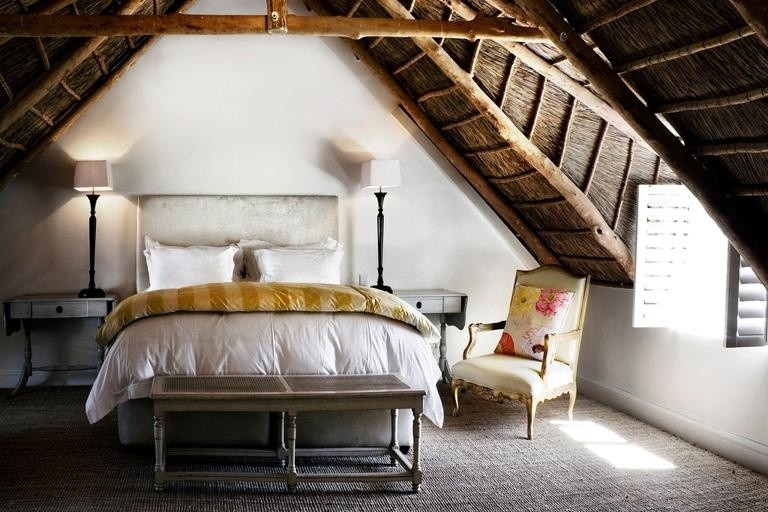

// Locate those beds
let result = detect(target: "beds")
[118,194,417,456]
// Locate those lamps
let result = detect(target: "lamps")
[71,161,115,298]
[360,159,403,295]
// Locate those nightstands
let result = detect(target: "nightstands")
[390,288,468,393]
[6,293,116,396]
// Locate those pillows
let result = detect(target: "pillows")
[142,234,344,291]
[493,280,577,366]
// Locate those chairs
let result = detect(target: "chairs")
[449,262,592,441]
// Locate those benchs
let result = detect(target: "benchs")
[149,374,427,494]
